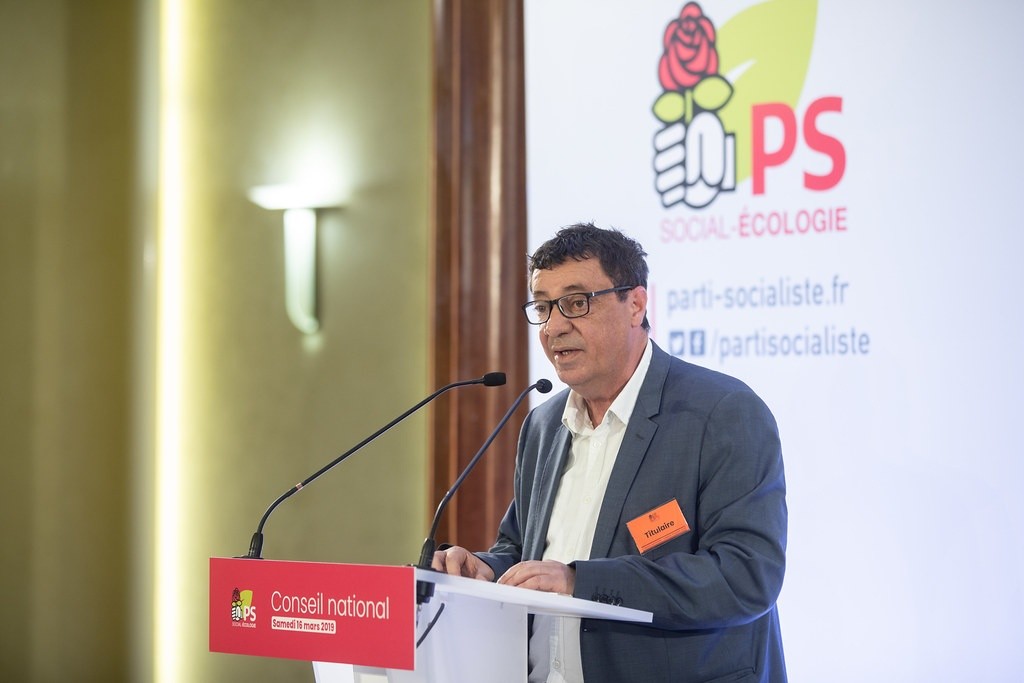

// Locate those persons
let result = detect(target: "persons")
[429,222,790,683]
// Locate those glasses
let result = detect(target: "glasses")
[522,286,632,323]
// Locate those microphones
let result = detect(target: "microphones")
[407,378,552,574]
[247,371,506,553]
